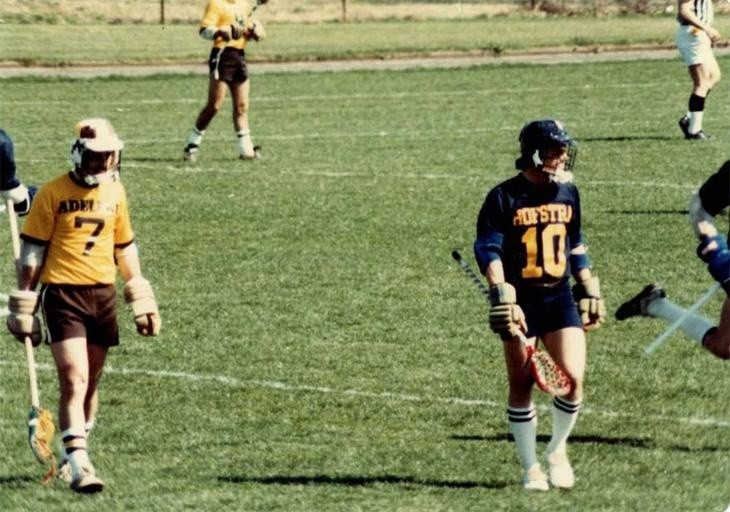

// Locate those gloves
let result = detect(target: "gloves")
[124,277,161,336]
[572,277,606,332]
[218,23,242,40]
[696,236,730,299]
[489,283,528,342]
[7,291,42,347]
[245,20,265,41]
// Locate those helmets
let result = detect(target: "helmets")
[515,119,578,183]
[70,118,123,184]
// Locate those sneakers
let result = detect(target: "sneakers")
[679,115,711,140]
[56,461,74,483]
[524,462,549,492]
[544,447,574,489]
[240,146,265,160]
[616,284,665,320]
[72,462,104,493]
[184,144,198,162]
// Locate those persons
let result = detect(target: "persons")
[615,160,730,360]
[473,118,607,493]
[675,0,723,142]
[0,128,38,218]
[183,0,266,162]
[6,117,162,494]
